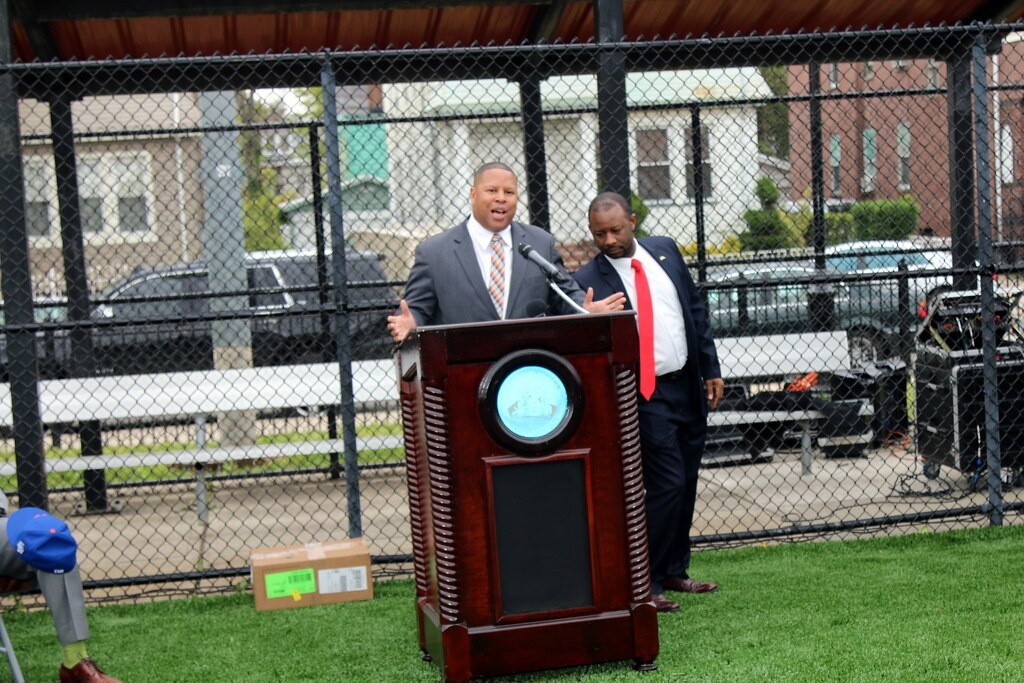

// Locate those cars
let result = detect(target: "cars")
[706,241,1001,371]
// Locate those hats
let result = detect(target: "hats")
[6,506,78,575]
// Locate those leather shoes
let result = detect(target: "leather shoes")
[59,656,122,683]
[669,570,718,593]
[652,595,681,613]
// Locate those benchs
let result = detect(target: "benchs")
[0,329,874,525]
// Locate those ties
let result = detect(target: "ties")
[629,259,657,401]
[487,233,508,321]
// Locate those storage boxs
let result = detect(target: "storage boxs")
[249,537,373,612]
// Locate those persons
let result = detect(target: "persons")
[0,489,122,683]
[570,193,723,612]
[387,163,626,342]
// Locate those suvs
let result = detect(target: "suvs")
[1,247,403,377]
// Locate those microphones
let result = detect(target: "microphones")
[517,242,564,280]
